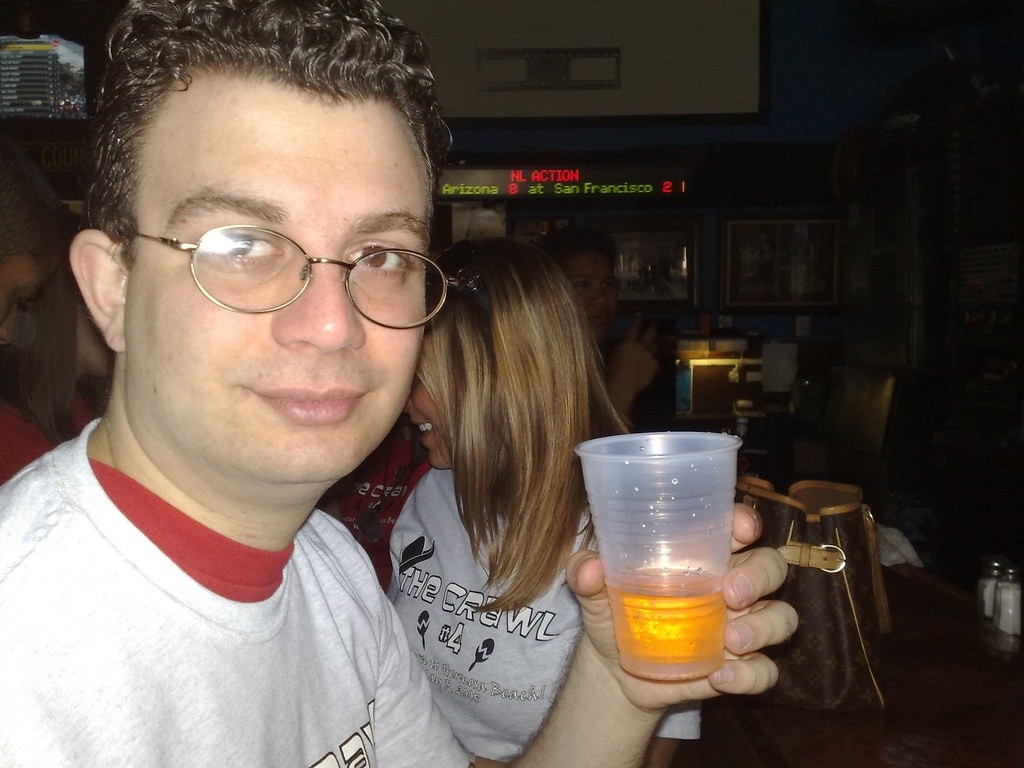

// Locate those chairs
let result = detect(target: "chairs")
[815,365,903,488]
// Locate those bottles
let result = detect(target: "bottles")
[978,562,1001,619]
[994,569,1024,640]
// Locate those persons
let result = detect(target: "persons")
[0,1,801,768]
[1,135,702,768]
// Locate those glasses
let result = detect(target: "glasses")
[112,223,477,330]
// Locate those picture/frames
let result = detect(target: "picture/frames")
[588,214,703,316]
[717,210,847,315]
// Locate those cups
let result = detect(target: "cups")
[574,432,743,679]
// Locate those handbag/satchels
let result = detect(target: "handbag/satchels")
[734,474,895,723]
